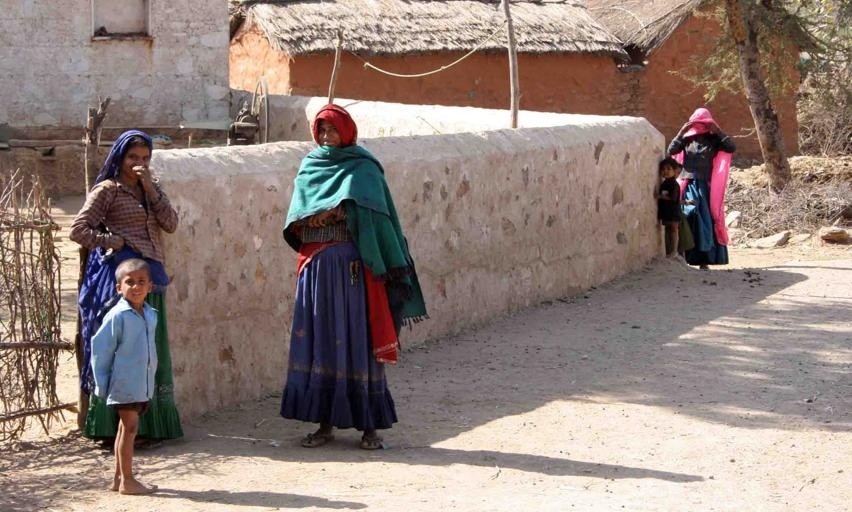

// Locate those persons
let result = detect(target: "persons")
[89,257,161,496]
[280,103,429,451]
[653,157,681,259]
[666,107,736,271]
[68,128,185,446]
[667,161,694,254]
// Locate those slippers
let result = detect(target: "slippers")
[360,435,382,449]
[302,429,334,447]
